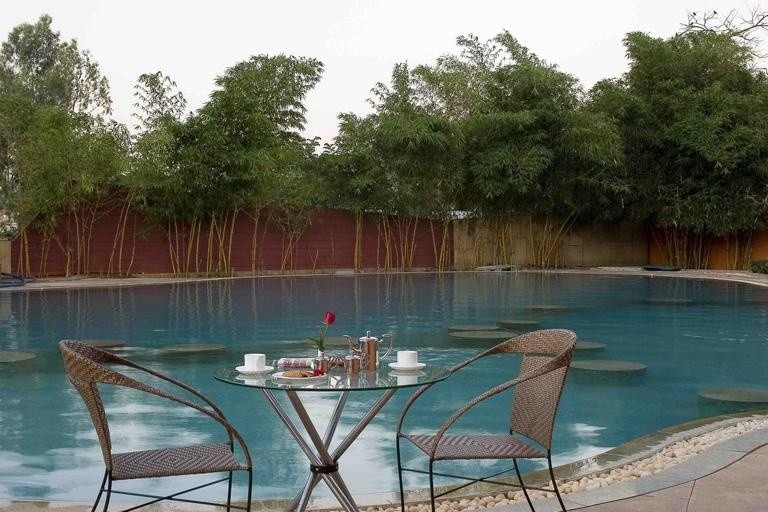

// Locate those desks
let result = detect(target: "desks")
[214,356,452,512]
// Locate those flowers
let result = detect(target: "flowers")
[309,309,337,349]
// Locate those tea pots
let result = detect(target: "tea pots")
[311,353,338,375]
[364,368,381,388]
[341,331,393,371]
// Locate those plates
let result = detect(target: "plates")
[387,372,427,378]
[271,378,328,386]
[233,364,275,378]
[269,370,329,380]
[235,373,275,382]
[388,362,426,372]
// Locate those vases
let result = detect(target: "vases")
[317,350,324,362]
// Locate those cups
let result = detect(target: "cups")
[243,378,269,389]
[345,370,361,388]
[397,350,418,366]
[397,376,419,386]
[242,353,267,370]
[343,354,361,376]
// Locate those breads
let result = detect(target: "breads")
[280,371,312,377]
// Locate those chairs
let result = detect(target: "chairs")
[397,327,579,512]
[58,338,255,512]
